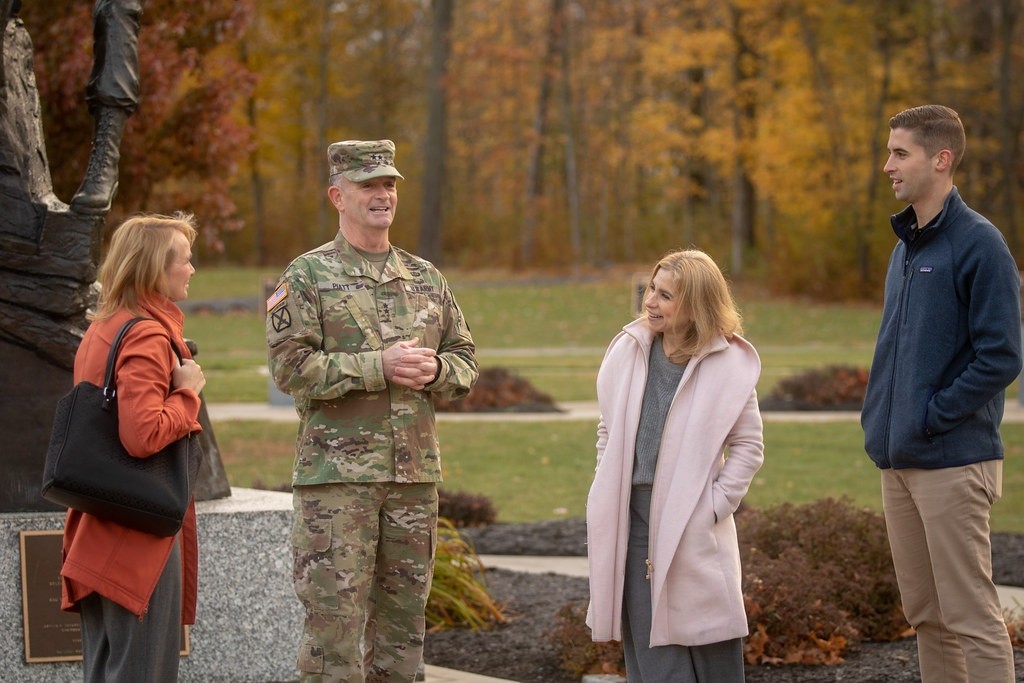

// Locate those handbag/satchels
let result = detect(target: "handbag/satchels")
[40,318,203,537]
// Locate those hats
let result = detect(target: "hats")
[327,140,404,182]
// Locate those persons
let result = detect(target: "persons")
[585,243,767,683]
[60,210,208,683]
[859,103,1023,683]
[264,138,480,683]
